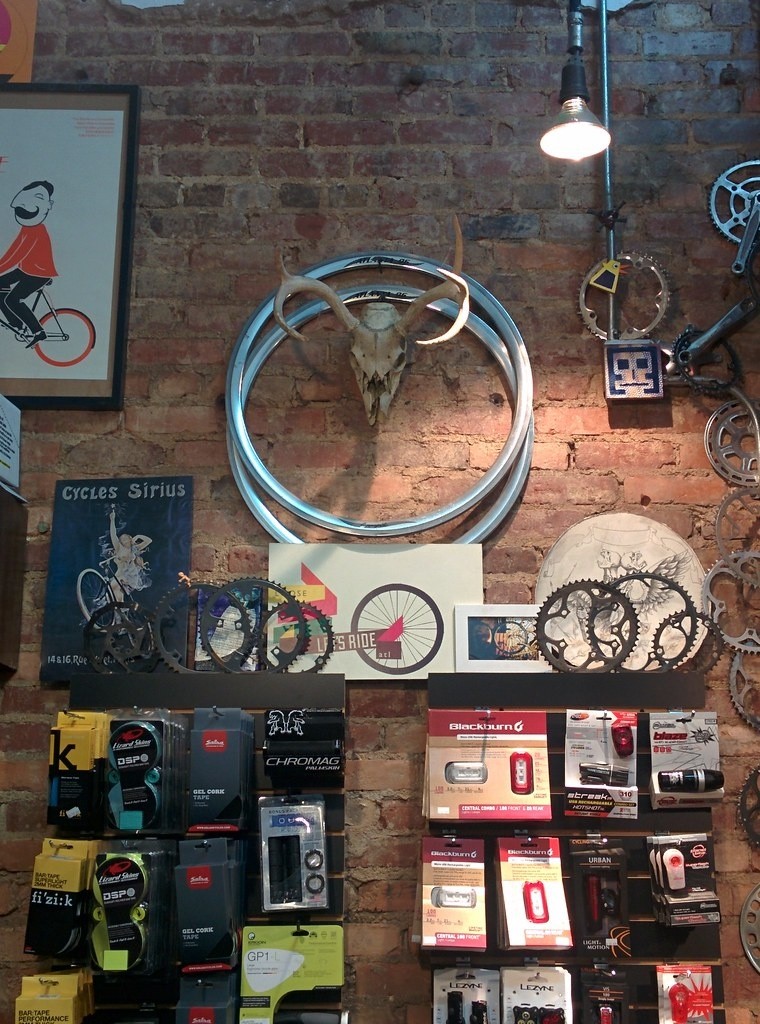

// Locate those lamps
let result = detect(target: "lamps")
[540,0,610,159]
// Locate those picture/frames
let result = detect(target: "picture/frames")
[454,604,552,673]
[0,80,143,411]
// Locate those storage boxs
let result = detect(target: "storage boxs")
[14,708,344,1024]
